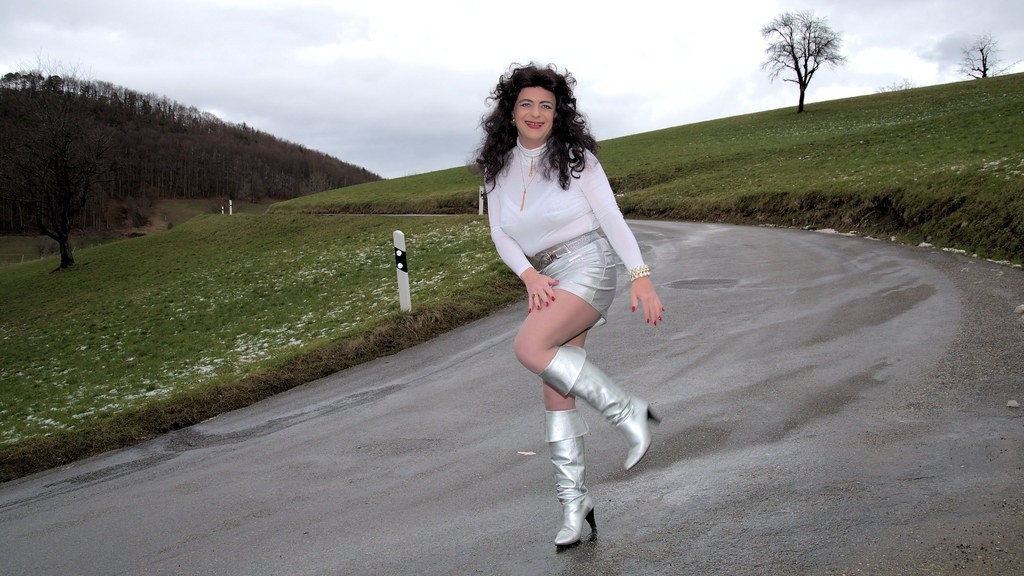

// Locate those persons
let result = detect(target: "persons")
[468,63,666,545]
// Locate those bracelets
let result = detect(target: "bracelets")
[628,265,650,282]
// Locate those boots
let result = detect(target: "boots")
[537,344,662,471]
[543,408,596,546]
